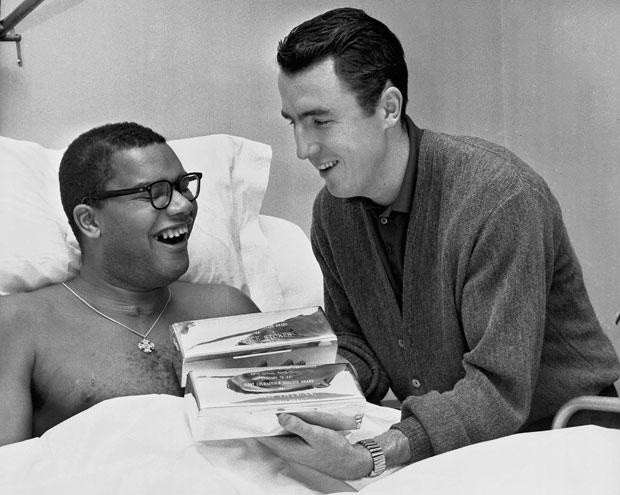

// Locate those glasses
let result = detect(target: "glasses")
[91,170,202,210]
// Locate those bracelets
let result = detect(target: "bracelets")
[358,438,387,479]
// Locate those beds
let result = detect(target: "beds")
[4,210,620,494]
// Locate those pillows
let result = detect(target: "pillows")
[2,129,281,317]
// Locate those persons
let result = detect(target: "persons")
[0,118,261,450]
[253,5,619,482]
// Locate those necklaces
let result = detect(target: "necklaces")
[61,280,174,355]
